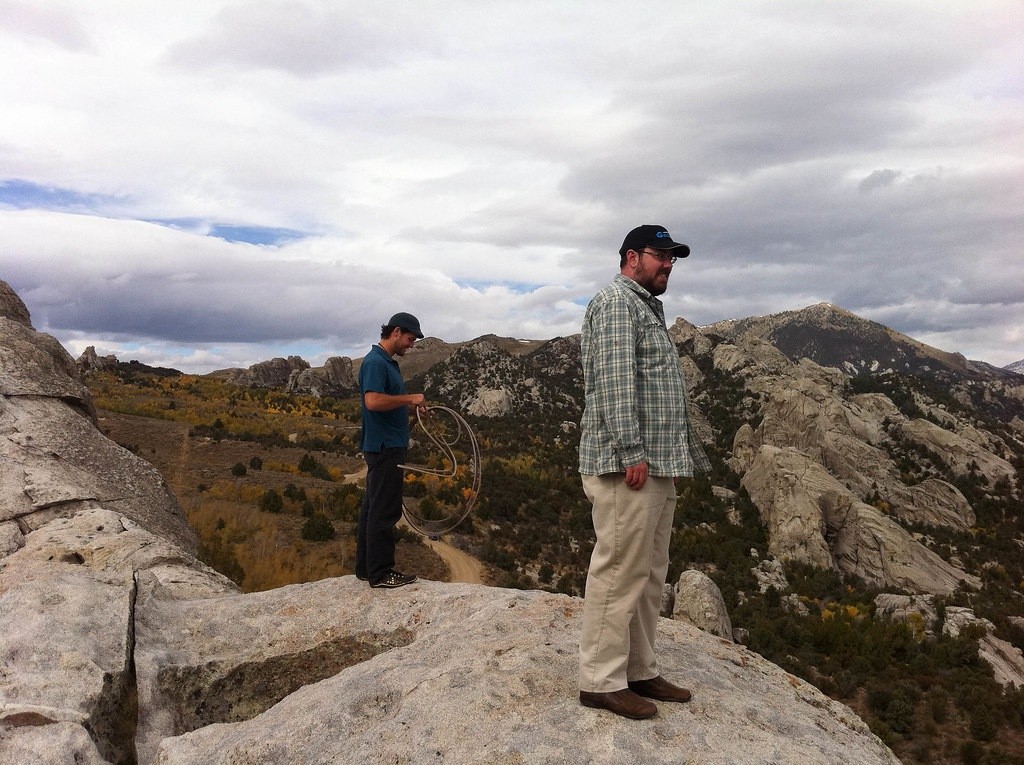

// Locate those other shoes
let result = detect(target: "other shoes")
[579,687,658,720]
[628,675,691,703]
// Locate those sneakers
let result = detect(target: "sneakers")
[355,571,370,579]
[370,569,418,588]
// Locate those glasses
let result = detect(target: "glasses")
[636,250,677,265]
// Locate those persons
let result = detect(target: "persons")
[578,224,712,720]
[355,312,426,588]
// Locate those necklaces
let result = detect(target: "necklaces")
[378,342,387,353]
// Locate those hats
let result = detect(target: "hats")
[619,224,690,258]
[388,312,424,338]
